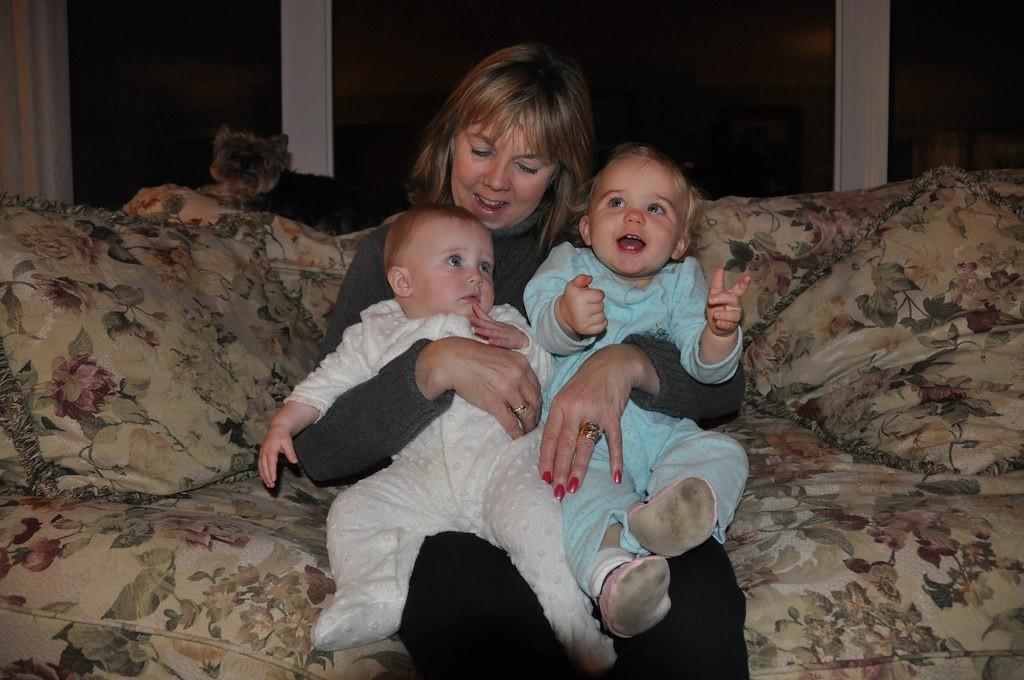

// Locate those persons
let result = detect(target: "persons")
[256,43,753,680]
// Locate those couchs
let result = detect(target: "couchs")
[0,184,1024,680]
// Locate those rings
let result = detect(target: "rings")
[578,420,605,444]
[512,399,530,416]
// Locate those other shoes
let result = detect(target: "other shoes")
[627,475,717,558]
[598,555,672,637]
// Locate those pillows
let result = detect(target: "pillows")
[738,166,1024,477]
[124,187,378,332]
[0,192,327,505]
